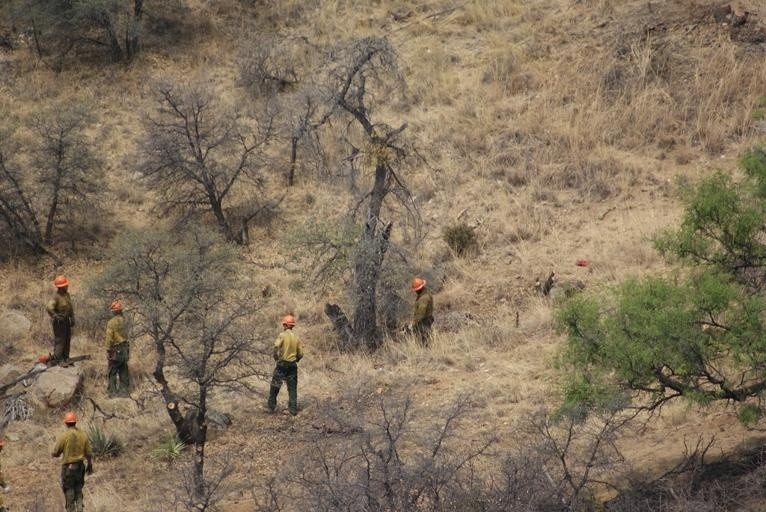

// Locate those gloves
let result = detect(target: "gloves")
[54,313,64,320]
[86,463,92,473]
[69,318,75,327]
[2,486,10,493]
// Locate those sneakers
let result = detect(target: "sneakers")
[58,358,73,368]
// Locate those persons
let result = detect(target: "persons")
[51,412,93,512]
[409,278,434,348]
[105,301,130,399]
[263,315,304,416]
[46,275,75,367]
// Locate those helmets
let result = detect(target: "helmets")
[52,276,70,288]
[282,315,296,325]
[110,300,123,311]
[411,277,426,291]
[64,412,76,423]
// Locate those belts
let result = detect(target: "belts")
[106,351,112,360]
[281,360,296,363]
[64,460,83,465]
